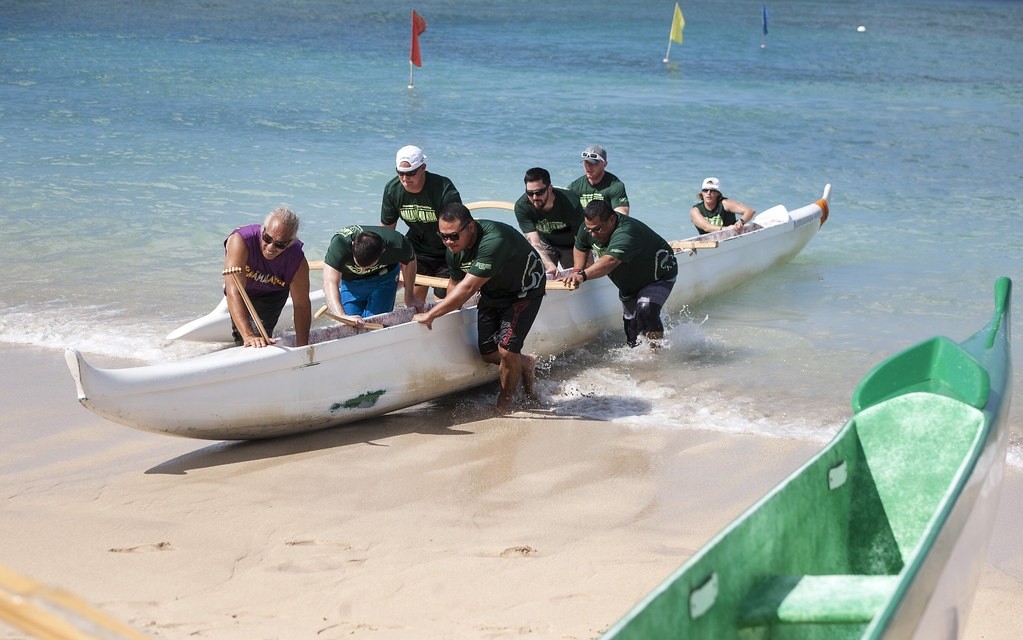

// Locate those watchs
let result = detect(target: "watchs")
[578,270,587,281]
[739,219,745,225]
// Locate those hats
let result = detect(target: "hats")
[702,177,725,198]
[580,145,607,163]
[395,145,428,172]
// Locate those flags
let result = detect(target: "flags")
[761,6,767,35]
[670,3,685,44]
[411,9,426,67]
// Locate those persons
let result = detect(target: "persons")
[558,200,678,344]
[223,208,311,347]
[412,203,545,404]
[567,145,630,257]
[380,146,462,305]
[324,225,423,331]
[514,167,587,280]
[691,176,756,235]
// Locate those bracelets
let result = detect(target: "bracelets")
[573,269,580,272]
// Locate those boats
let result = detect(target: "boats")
[165,201,515,348]
[61,181,837,445]
[592,271,1017,640]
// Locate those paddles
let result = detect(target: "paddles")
[314,303,387,331]
[221,266,273,346]
[753,204,790,226]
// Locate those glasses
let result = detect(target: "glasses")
[526,184,550,197]
[262,226,292,249]
[436,220,472,241]
[581,152,605,163]
[396,164,423,176]
[586,220,609,232]
[701,188,719,193]
